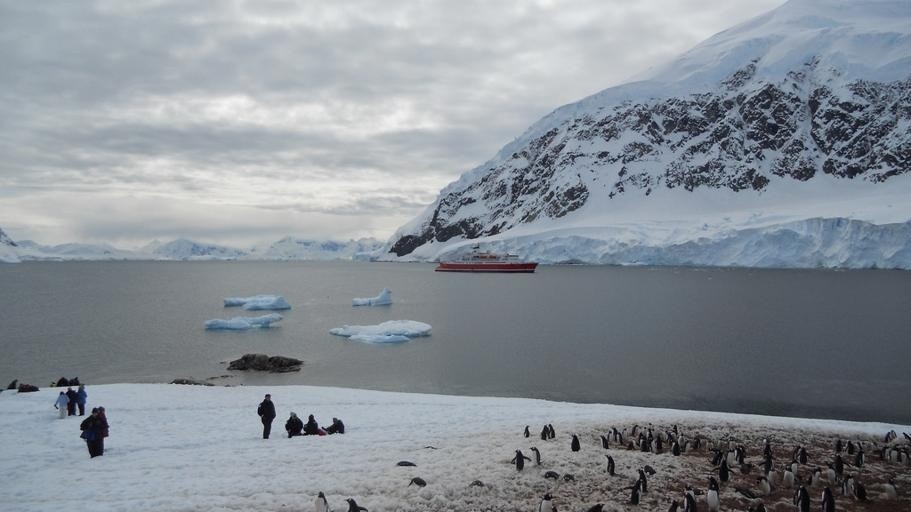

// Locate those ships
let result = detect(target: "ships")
[433,242,539,273]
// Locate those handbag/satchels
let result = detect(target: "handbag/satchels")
[80,431,95,440]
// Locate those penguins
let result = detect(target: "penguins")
[344,497,369,512]
[314,491,331,512]
[408,477,426,486]
[507,424,911,512]
[397,461,418,466]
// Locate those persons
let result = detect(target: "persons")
[55,386,109,458]
[258,394,276,439]
[285,412,344,438]
